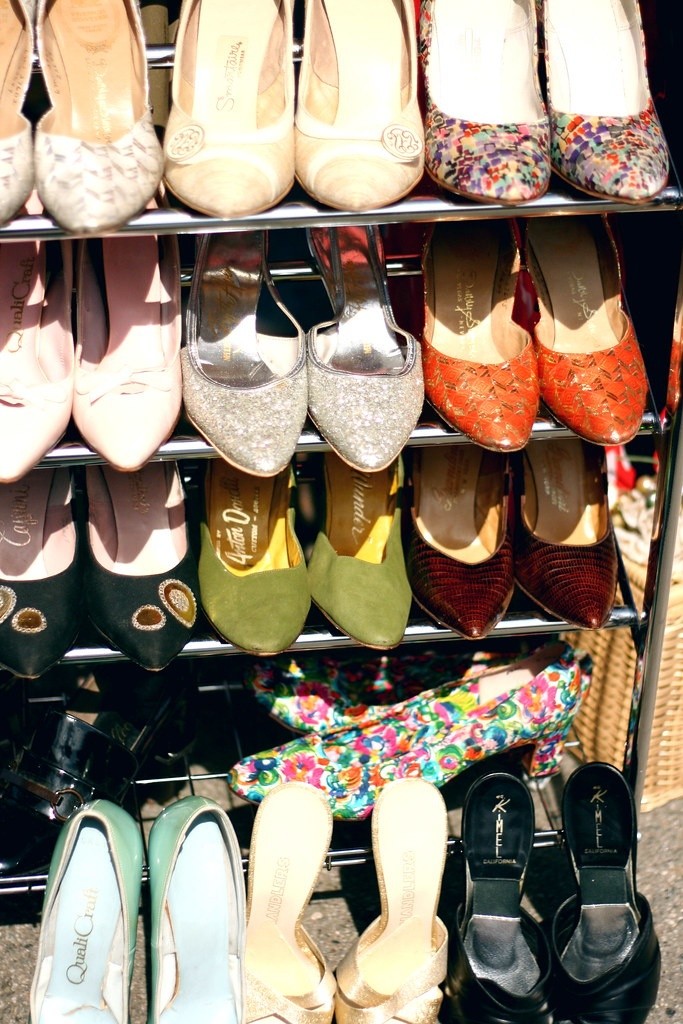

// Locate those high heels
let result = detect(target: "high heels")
[424,0,667,203]
[0,645,660,1023]
[0,235,183,482]
[182,226,424,478]
[424,213,648,452]
[1,0,163,233]
[162,0,423,216]
[0,436,618,678]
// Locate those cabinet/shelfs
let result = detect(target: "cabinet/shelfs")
[0,45,683,895]
